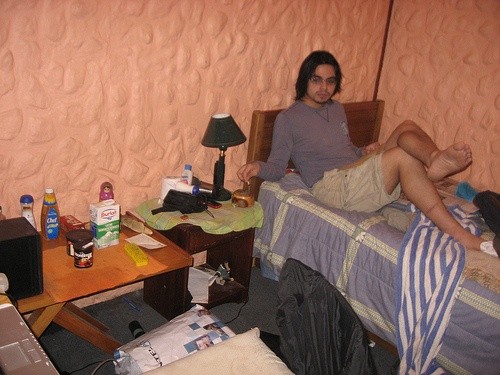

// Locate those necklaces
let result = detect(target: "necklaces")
[309,102,330,124]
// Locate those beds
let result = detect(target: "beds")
[243,100,500,375]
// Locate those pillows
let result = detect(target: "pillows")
[140,327,296,375]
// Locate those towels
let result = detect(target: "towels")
[393,203,485,375]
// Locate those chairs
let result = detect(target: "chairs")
[273,258,401,375]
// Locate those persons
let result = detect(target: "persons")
[196,306,228,352]
[237,50,488,251]
[99,182,114,203]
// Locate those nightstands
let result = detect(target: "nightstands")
[126,180,265,322]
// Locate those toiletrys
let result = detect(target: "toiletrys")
[183,163,195,188]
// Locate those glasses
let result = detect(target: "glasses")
[309,76,338,86]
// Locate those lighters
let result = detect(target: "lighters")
[207,200,222,208]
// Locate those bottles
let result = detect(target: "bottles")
[181,164,193,186]
[41,188,60,242]
[20,195,37,230]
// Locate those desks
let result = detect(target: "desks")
[15,211,194,352]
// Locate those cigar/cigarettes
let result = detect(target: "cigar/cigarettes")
[247,180,250,186]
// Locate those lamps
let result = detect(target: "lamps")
[201,113,247,201]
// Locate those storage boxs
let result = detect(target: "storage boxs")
[0,217,43,300]
